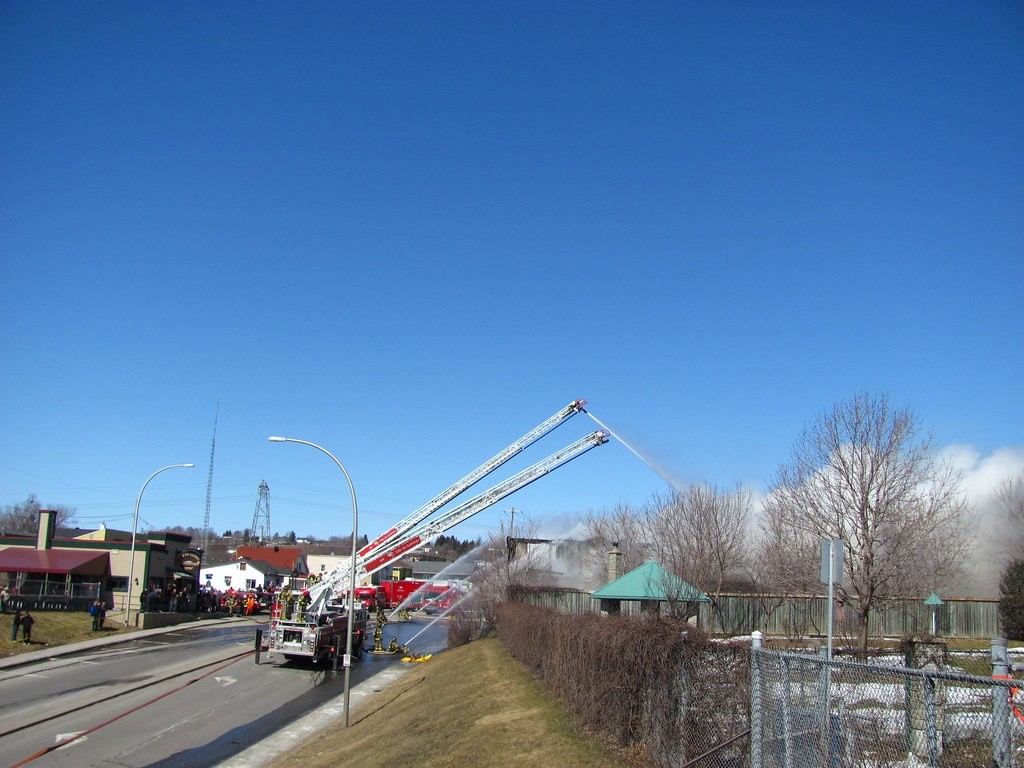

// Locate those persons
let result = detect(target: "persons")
[397,601,412,620]
[307,573,322,586]
[375,600,387,626]
[390,637,399,652]
[1,587,10,612]
[12,611,34,645]
[139,583,276,618]
[90,601,107,631]
[279,585,311,623]
[374,624,384,652]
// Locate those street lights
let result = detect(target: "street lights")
[125,464,195,626]
[268,436,358,728]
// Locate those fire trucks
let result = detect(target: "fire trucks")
[272,572,494,616]
[256,402,611,671]
